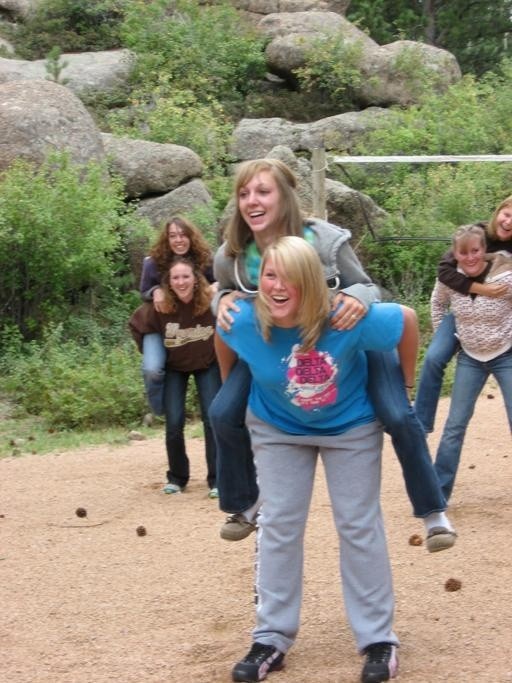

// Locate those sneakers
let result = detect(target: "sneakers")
[232,642,284,682]
[162,484,178,492]
[361,642,398,683]
[220,514,257,541]
[426,527,456,553]
[209,488,218,498]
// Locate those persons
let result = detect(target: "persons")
[425,225,510,516]
[412,195,512,434]
[137,214,236,415]
[214,233,418,683]
[215,154,457,553]
[128,257,228,499]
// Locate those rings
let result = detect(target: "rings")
[347,313,359,322]
